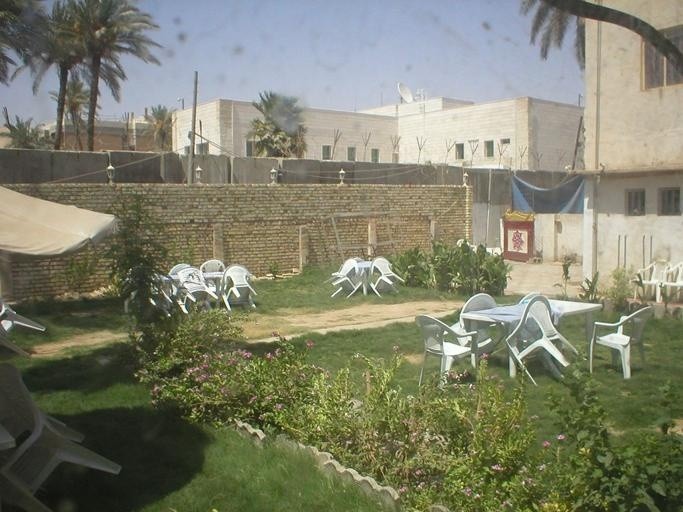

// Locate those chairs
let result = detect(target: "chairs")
[588,304,654,379]
[505,295,587,387]
[414,314,478,387]
[324,256,407,299]
[152,260,259,316]
[0,296,123,512]
[489,292,541,346]
[633,258,682,304]
[437,292,506,351]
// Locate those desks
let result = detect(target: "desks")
[461,299,603,378]
